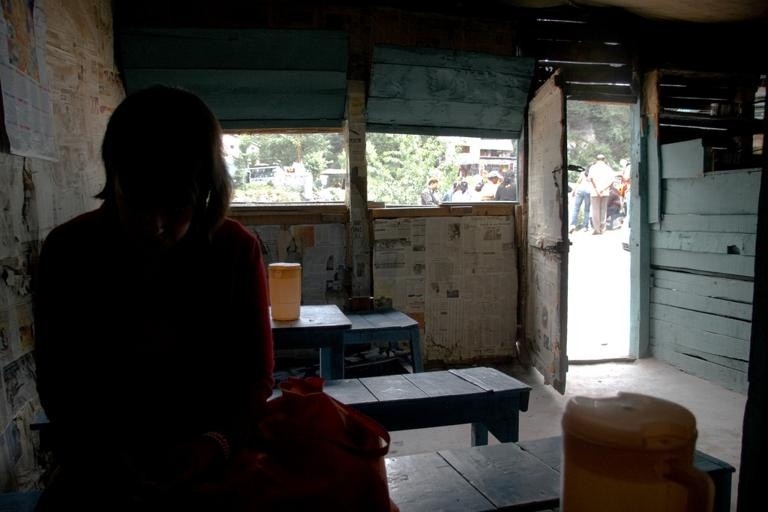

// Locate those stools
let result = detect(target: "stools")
[344,307,424,378]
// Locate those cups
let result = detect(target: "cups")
[560,389,716,512]
[268,262,302,321]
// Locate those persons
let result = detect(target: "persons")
[420,153,630,237]
[30,85,274,511]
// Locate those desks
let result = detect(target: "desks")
[268,303,352,380]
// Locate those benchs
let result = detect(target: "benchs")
[382,429,736,512]
[272,365,533,446]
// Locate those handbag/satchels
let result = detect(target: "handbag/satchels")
[225,378,398,512]
